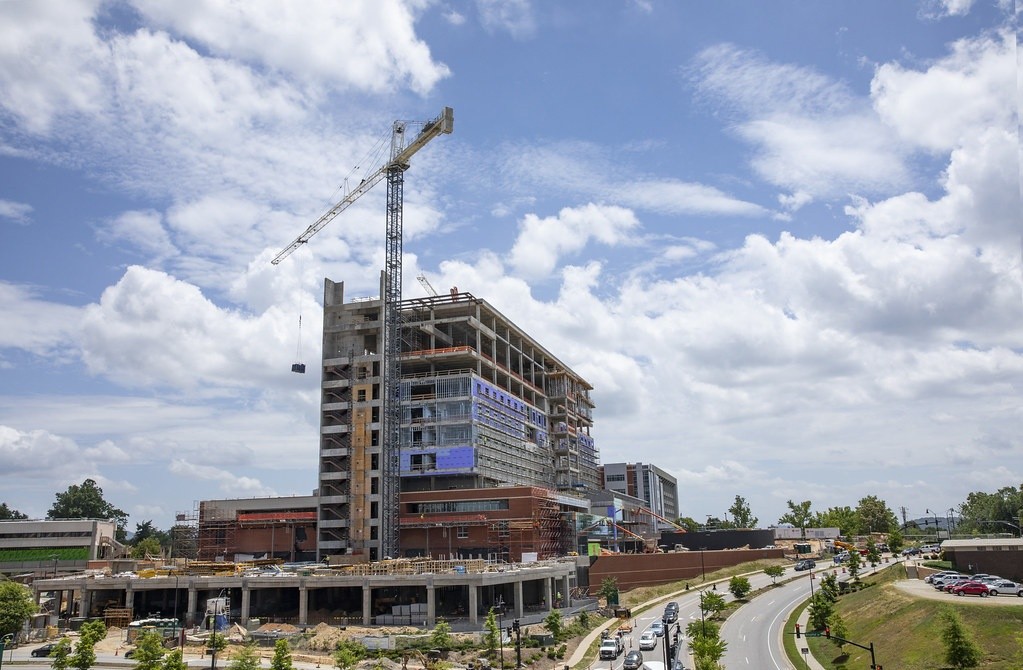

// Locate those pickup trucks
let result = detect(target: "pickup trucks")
[920,545,938,553]
[837,550,861,562]
[986,581,1023,597]
[858,549,869,556]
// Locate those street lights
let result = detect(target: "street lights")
[707,515,712,527]
[53,557,58,581]
[212,587,230,669]
[724,513,727,521]
[947,508,954,540]
[500,595,507,670]
[927,509,940,544]
[168,569,178,640]
[685,582,718,638]
[810,573,815,610]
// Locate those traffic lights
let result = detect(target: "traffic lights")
[507,628,513,638]
[878,665,882,670]
[513,622,517,631]
[825,627,831,639]
[795,624,800,639]
[516,620,519,630]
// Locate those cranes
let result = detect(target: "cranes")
[267,102,454,561]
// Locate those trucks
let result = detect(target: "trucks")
[598,636,626,659]
[659,543,690,552]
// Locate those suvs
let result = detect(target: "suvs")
[933,574,970,584]
[975,577,1001,583]
[954,582,989,597]
[970,574,989,579]
[624,650,643,669]
[925,571,959,582]
[638,630,657,650]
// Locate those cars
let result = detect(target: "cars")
[876,544,890,552]
[31,644,72,659]
[943,579,977,594]
[650,621,666,636]
[902,548,922,555]
[982,579,1010,584]
[935,581,954,591]
[662,611,677,623]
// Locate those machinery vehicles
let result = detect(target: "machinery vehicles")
[402,647,442,670]
[632,506,688,534]
[834,540,869,556]
[607,520,663,554]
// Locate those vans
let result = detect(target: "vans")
[795,559,815,571]
[664,602,680,613]
